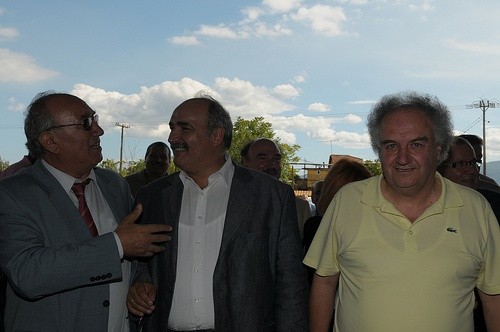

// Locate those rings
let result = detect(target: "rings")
[129,299,134,303]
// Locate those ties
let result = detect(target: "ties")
[72,178,99,238]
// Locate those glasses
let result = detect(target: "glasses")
[38,114,98,135]
[441,159,482,169]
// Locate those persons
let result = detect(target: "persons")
[0,89,173,332]
[240,138,311,240]
[437,135,500,226]
[0,151,37,179]
[301,90,500,332]
[301,158,372,270]
[124,141,171,199]
[125,90,311,332]
